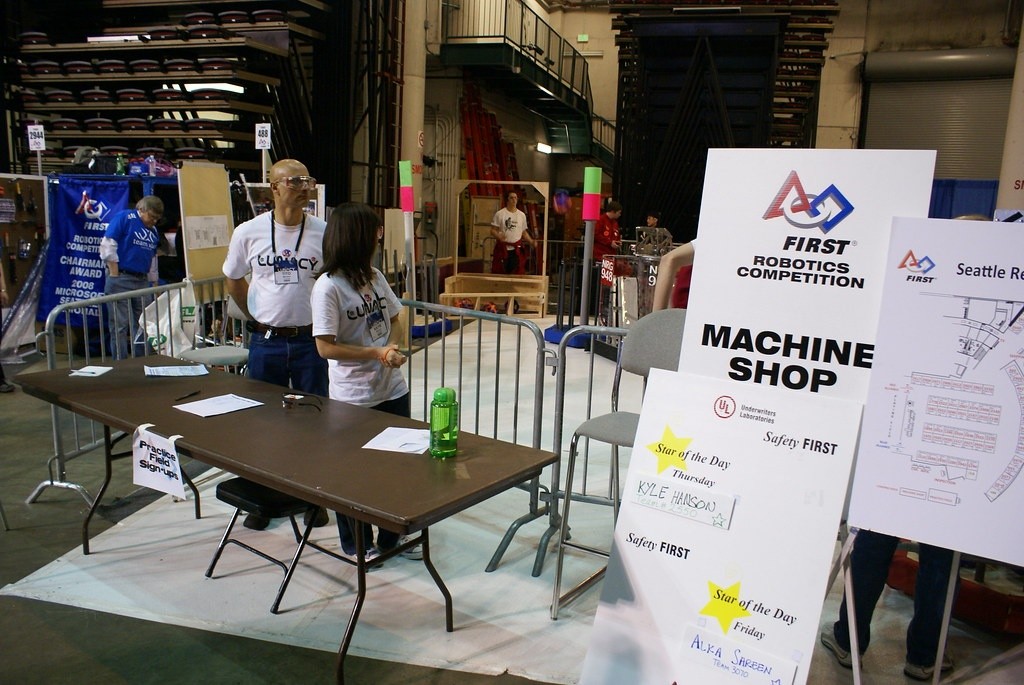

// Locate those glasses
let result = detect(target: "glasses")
[273,176,317,190]
[143,209,162,222]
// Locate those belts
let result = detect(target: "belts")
[255,323,313,337]
[119,269,148,278]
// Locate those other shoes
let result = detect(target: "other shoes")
[0,383,15,392]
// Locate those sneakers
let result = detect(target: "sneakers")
[904,649,955,680]
[822,620,863,668]
[353,547,384,566]
[244,513,270,529]
[303,508,330,527]
[396,535,423,559]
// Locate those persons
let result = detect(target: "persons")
[223,159,329,531]
[589,201,622,315]
[490,192,537,276]
[638,212,665,244]
[176,225,221,340]
[99,196,165,361]
[652,238,697,312]
[310,202,422,568]
[821,215,993,682]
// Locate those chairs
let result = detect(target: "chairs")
[549,307,687,620]
[179,295,251,376]
[204,476,324,613]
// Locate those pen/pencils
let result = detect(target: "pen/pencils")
[70,370,95,374]
[175,390,201,401]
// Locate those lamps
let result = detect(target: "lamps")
[545,57,555,67]
[521,43,545,56]
[537,142,552,155]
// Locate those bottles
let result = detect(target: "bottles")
[0,179,46,285]
[429,388,459,459]
[116,151,158,178]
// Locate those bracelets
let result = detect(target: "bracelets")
[383,348,393,360]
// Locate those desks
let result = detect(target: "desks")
[9,355,558,685]
